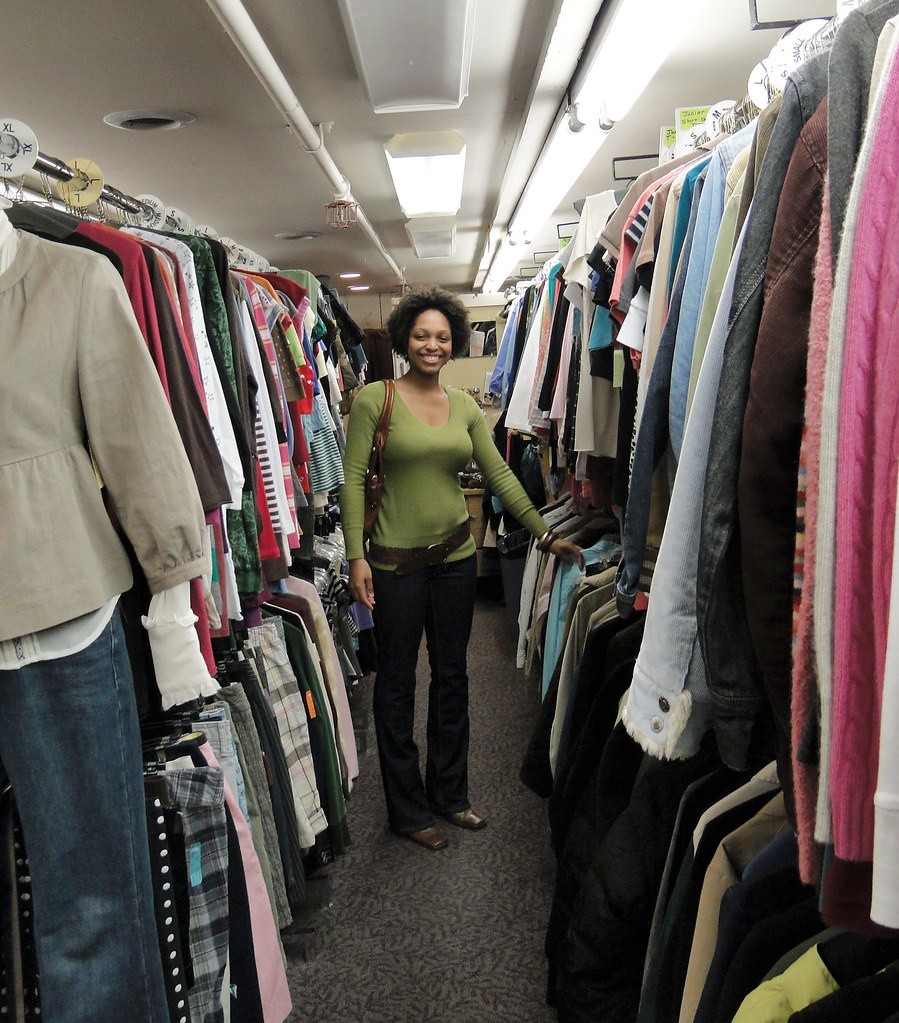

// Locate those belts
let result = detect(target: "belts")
[369,517,470,567]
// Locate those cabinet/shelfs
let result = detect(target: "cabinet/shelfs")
[461,488,497,578]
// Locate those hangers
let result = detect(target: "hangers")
[691,9,846,148]
[1,133,270,272]
[142,527,346,774]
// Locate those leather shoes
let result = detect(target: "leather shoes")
[440,808,486,830]
[406,822,448,850]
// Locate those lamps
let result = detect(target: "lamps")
[336,1,691,295]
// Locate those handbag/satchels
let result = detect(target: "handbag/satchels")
[363,379,395,542]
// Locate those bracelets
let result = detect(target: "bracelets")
[536,529,559,554]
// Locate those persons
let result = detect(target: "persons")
[341,287,586,853]
